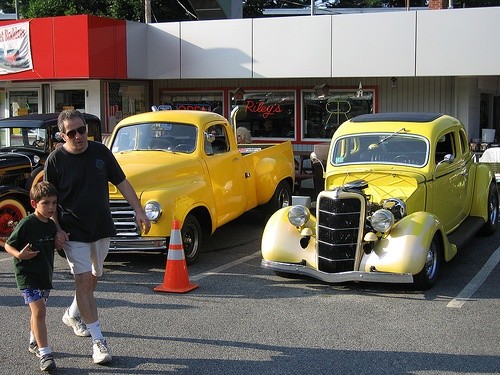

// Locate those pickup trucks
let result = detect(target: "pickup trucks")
[58,109,295,268]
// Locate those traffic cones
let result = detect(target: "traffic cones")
[153,217,200,293]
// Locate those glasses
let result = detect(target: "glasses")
[63,126,86,138]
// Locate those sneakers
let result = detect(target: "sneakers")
[39,353,56,371]
[29,341,40,358]
[62,307,91,336]
[92,337,112,363]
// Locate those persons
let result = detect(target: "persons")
[5,181,66,371]
[210,129,218,135]
[45,110,151,365]
[237,127,253,151]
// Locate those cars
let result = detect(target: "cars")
[0,111,104,252]
[261,112,500,291]
